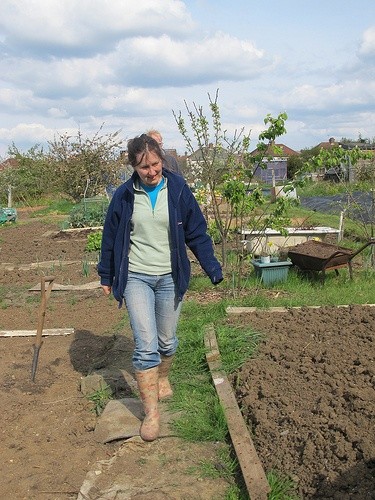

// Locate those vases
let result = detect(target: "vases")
[270,257,278,263]
[261,256,271,264]
[249,257,293,285]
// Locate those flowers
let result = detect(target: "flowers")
[261,240,274,257]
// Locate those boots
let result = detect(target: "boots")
[158,351,176,401]
[135,365,160,442]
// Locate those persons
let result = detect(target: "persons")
[95,132,224,444]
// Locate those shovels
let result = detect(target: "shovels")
[28,275,57,381]
